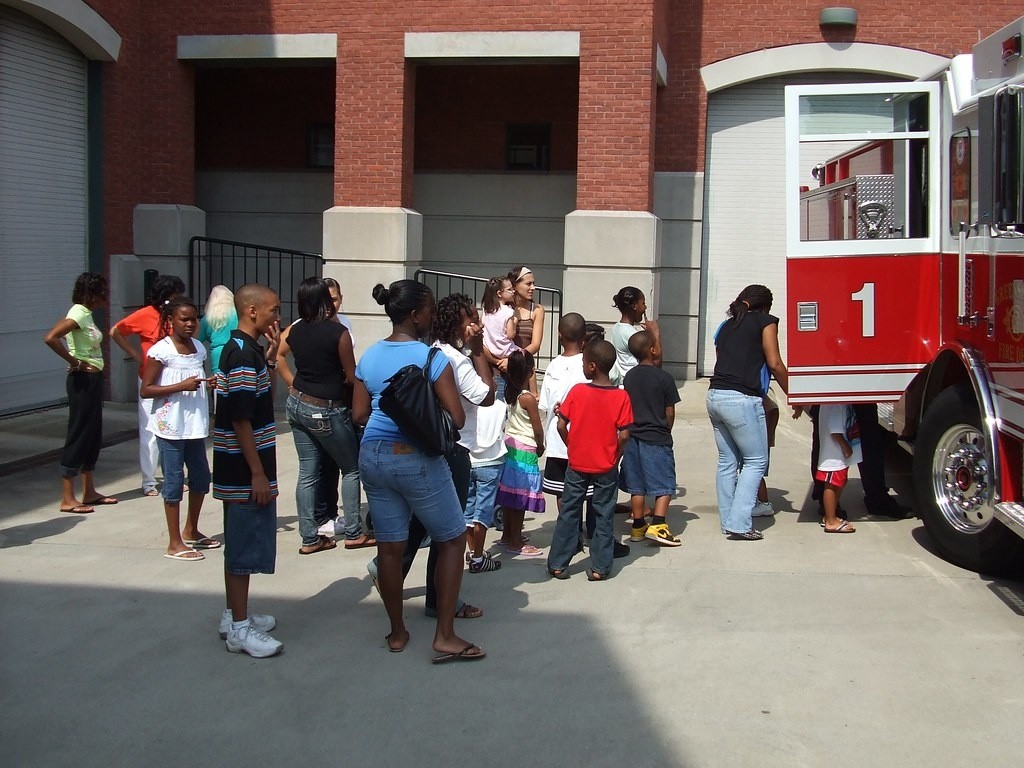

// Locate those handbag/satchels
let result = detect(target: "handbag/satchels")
[379,362,460,457]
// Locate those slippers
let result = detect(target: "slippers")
[387,630,410,653]
[183,537,221,549]
[819,517,856,533]
[142,485,158,496]
[344,535,378,549]
[82,496,118,505]
[164,547,205,561]
[456,604,484,618]
[430,642,486,664]
[497,536,543,557]
[298,535,337,554]
[59,503,93,514]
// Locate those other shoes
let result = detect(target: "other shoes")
[585,567,602,581]
[547,566,568,579]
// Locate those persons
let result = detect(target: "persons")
[803,402,914,533]
[538,286,662,559]
[548,340,633,581]
[203,285,239,437]
[706,285,803,539]
[353,278,486,663]
[276,276,377,554]
[481,265,548,419]
[45,272,117,513]
[140,296,220,561]
[109,275,199,496]
[618,331,681,547]
[368,293,546,619]
[214,284,284,658]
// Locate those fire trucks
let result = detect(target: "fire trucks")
[785,13,1024,572]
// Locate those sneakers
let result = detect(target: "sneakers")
[731,527,763,540]
[613,541,630,558]
[226,620,284,658]
[630,521,683,546]
[466,550,501,573]
[317,520,338,536]
[868,493,912,518]
[749,501,775,517]
[219,611,276,640]
[817,503,847,522]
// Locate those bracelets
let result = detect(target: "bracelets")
[287,386,293,389]
[73,360,82,371]
